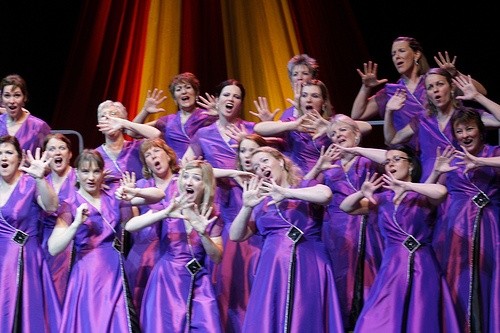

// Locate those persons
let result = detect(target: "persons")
[339,147,460,333]
[48,149,166,333]
[425,107,500,333]
[0,136,62,333]
[229,146,344,333]
[0,35,500,333]
[124,159,225,333]
